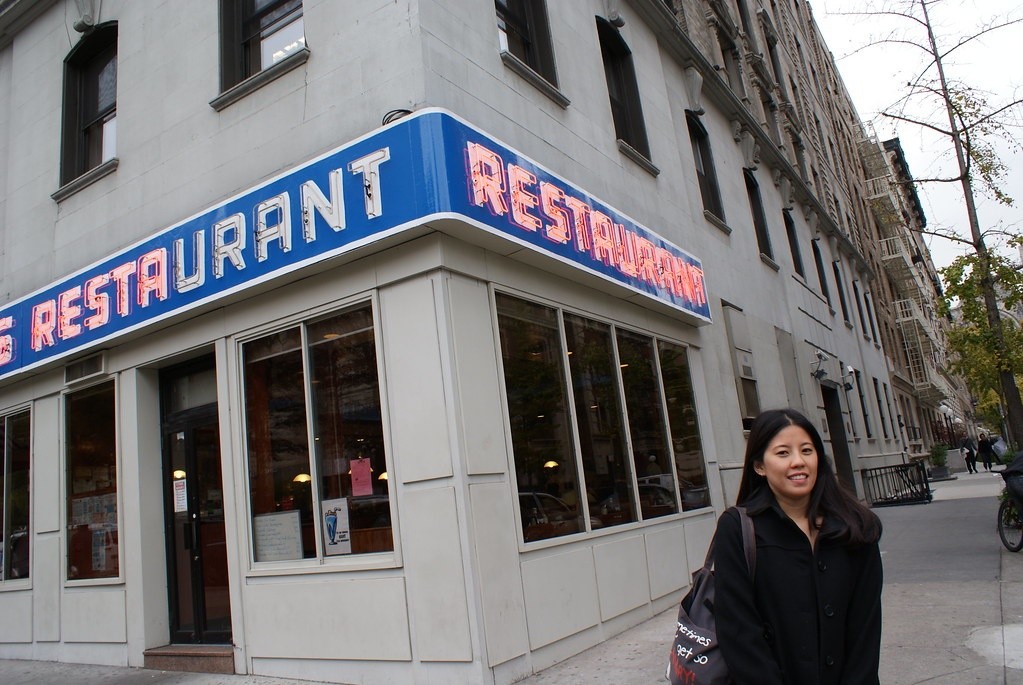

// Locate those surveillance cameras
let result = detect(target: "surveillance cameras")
[847,365,854,373]
[815,349,830,361]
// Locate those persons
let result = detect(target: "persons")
[665,408,884,685]
[960,431,978,474]
[543,480,579,512]
[978,434,992,472]
[646,455,662,506]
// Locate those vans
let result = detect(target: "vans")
[9,523,119,577]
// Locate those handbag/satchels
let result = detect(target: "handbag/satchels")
[664,507,757,685]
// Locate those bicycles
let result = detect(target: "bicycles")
[998,456,1023,552]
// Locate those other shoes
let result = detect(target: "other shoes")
[974,469,978,473]
[970,469,972,474]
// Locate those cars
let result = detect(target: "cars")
[601,483,698,526]
[637,474,712,508]
[518,492,606,543]
